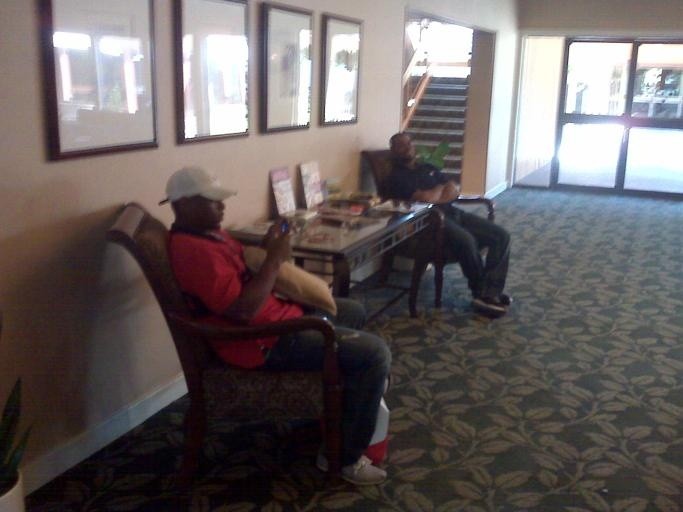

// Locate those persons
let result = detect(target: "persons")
[380,133,514,314]
[158,165,390,487]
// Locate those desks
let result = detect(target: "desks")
[223,188,438,333]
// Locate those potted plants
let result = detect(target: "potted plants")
[0,369,39,512]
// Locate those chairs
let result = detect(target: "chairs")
[356,147,499,309]
[92,200,352,480]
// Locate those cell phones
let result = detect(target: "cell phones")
[281,219,289,233]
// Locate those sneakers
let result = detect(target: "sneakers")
[317,451,389,487]
[469,285,515,315]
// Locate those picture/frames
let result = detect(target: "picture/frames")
[170,1,250,146]
[35,0,160,165]
[319,11,363,127]
[258,0,312,135]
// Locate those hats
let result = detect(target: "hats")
[159,164,239,204]
